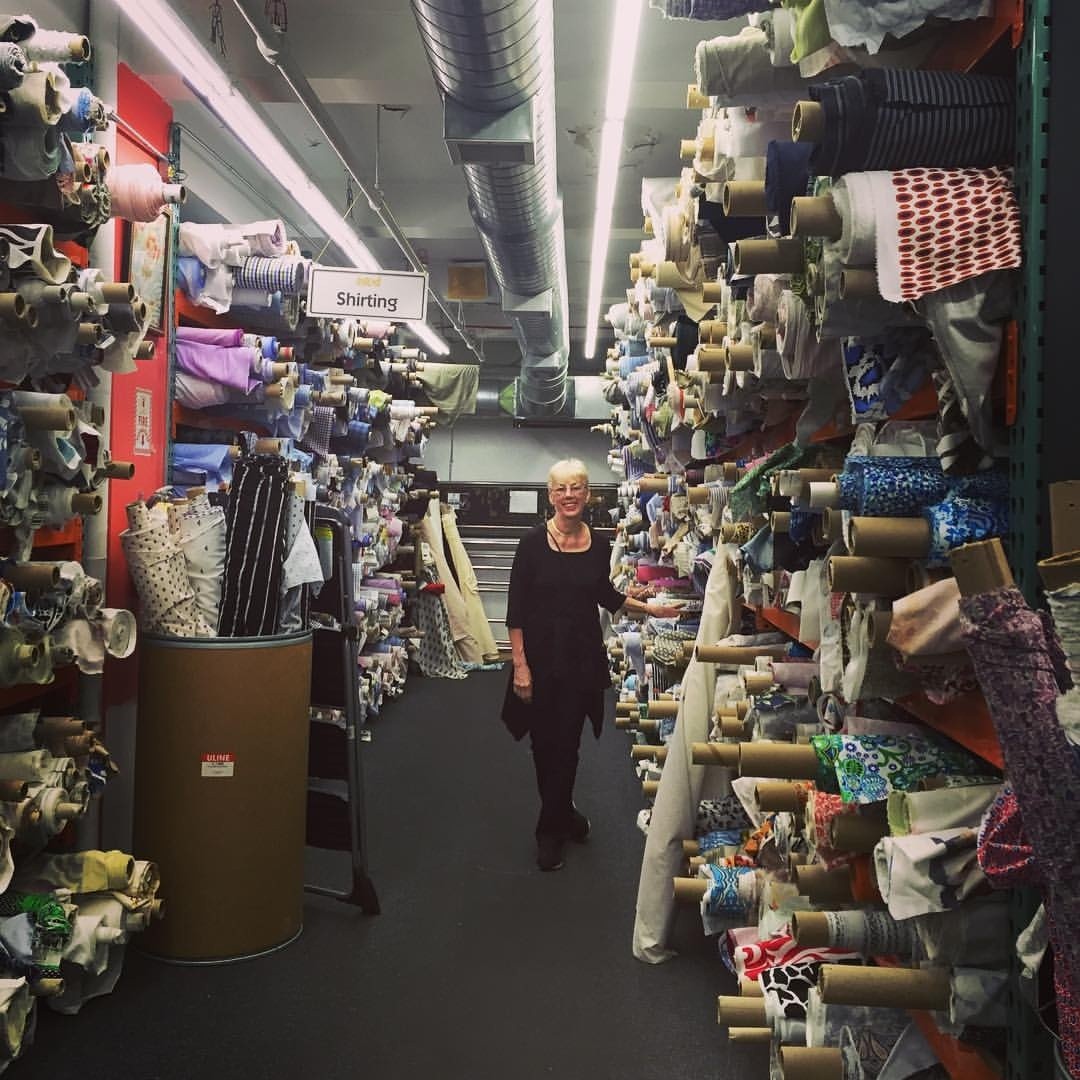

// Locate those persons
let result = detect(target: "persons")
[500,459,688,872]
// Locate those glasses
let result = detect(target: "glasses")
[549,484,588,497]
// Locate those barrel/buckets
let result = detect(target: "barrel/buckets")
[131,622,312,965]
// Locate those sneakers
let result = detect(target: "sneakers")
[535,833,567,870]
[564,799,592,842]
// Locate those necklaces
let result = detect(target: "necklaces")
[549,516,583,537]
